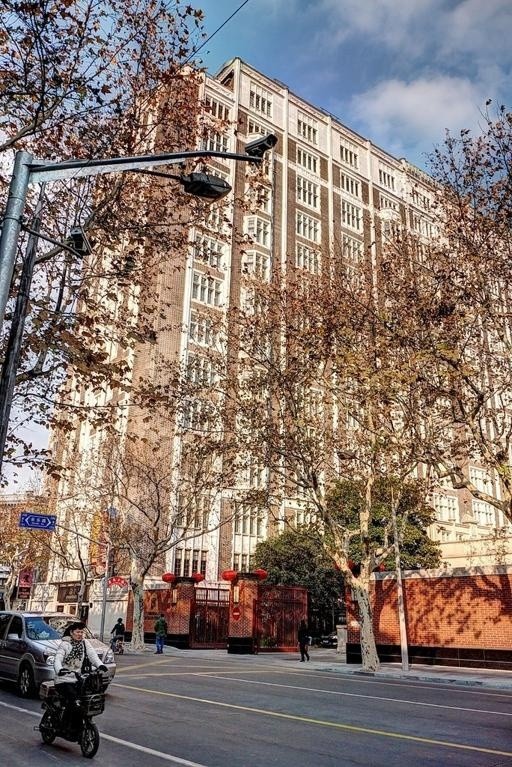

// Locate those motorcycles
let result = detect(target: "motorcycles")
[39,669,105,757]
[110,632,124,655]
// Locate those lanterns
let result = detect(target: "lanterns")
[222,569,237,581]
[254,569,268,581]
[376,563,386,573]
[333,560,355,572]
[162,573,176,583]
[191,573,204,585]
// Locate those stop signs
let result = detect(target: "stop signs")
[232,606,241,621]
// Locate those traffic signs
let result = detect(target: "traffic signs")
[20,512,57,530]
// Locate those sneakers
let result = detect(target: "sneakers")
[153,650,163,654]
[300,656,310,662]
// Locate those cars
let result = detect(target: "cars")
[321,630,337,648]
[0,610,116,698]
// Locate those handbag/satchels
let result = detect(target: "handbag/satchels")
[81,655,93,674]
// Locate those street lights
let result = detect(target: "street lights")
[337,449,408,672]
[0,167,233,478]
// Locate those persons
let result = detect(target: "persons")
[111,618,126,651]
[154,614,168,654]
[297,623,310,662]
[52,623,108,740]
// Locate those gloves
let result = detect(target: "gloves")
[99,665,108,672]
[58,668,71,676]
